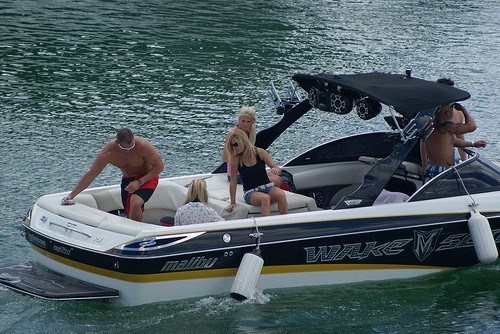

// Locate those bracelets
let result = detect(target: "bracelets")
[471,141,475,146]
[232,203,236,205]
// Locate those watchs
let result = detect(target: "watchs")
[137,178,143,186]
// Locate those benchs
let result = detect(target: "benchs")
[206,186,308,218]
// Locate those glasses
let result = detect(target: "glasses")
[118,139,135,151]
[231,142,238,146]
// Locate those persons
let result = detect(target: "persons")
[62,128,163,222]
[174,179,226,226]
[222,107,282,188]
[225,130,289,217]
[419,78,487,194]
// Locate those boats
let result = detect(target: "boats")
[1,68,500,308]
